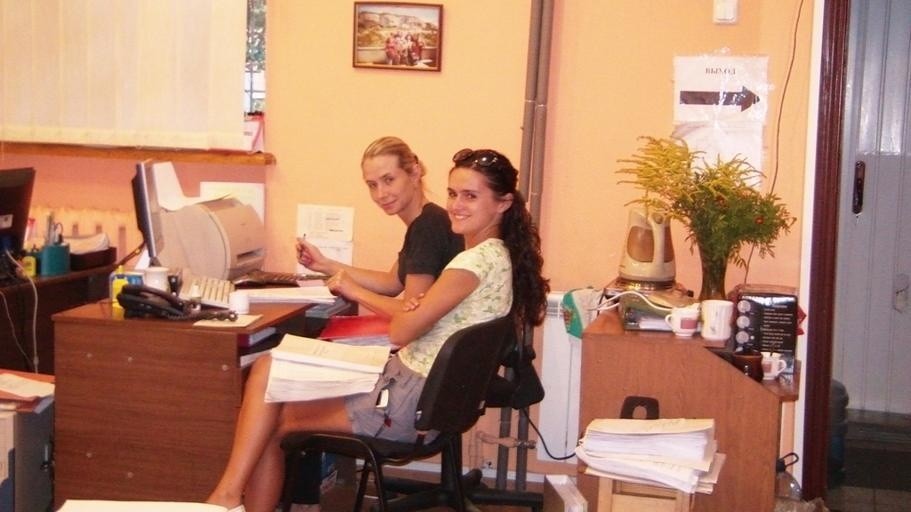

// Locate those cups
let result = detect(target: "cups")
[762,351,787,380]
[228,291,249,314]
[143,266,170,296]
[700,299,734,340]
[664,307,700,337]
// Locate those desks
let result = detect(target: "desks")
[0,262,119,374]
[48,288,322,512]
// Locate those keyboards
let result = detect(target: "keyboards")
[179,273,236,309]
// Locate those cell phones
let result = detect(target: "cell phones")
[376,388,390,408]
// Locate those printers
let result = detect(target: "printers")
[162,197,268,282]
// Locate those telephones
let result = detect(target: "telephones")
[116,284,187,318]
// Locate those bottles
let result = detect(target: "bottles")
[112,265,128,308]
[191,284,202,310]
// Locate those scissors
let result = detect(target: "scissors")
[50,222,63,245]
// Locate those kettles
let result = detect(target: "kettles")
[618,203,679,285]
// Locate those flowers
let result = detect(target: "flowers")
[607,128,803,276]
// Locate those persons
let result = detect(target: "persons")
[206,147,550,511]
[382,30,424,64]
[292,135,465,317]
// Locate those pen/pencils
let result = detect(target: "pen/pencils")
[45,212,55,244]
[300,233,306,258]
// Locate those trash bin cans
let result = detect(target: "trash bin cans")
[828,379,850,481]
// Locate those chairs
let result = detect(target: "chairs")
[372,281,546,512]
[279,314,512,511]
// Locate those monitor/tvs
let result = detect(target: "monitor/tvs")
[115,158,168,270]
[1,166,36,286]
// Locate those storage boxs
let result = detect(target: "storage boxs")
[2,394,56,512]
[0,407,21,512]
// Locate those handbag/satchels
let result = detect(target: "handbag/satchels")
[486,310,545,409]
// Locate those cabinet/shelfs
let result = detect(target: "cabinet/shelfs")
[577,298,798,510]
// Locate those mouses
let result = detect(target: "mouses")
[231,275,266,287]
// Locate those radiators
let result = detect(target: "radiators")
[534,290,584,464]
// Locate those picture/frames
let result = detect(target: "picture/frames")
[351,1,448,72]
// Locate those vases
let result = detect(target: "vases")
[692,244,728,301]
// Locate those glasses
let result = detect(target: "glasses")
[452,149,498,166]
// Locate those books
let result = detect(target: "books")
[542,413,725,511]
[615,293,703,333]
[234,285,393,405]
[64,231,111,254]
[0,363,56,417]
